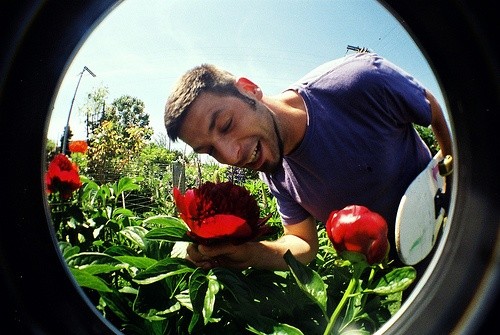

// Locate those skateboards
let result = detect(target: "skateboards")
[393,146,455,266]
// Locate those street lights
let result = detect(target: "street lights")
[59,66,96,156]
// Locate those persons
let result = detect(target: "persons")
[163,51,453,271]
